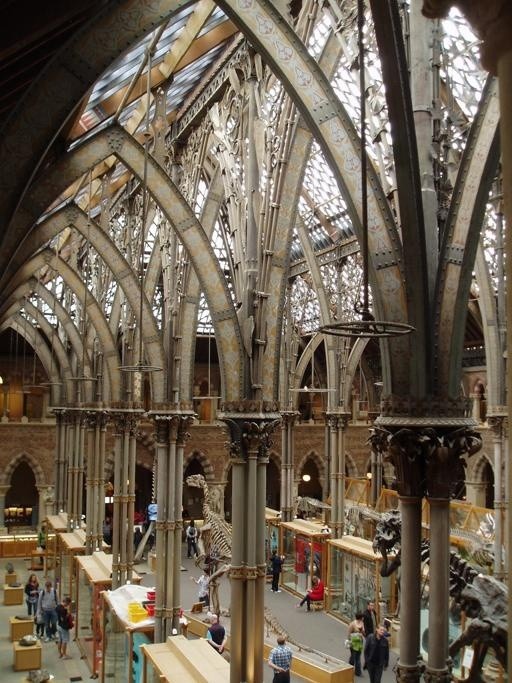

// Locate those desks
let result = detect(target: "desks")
[3,568,25,605]
[30,548,54,571]
[9,615,42,670]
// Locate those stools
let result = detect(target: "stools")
[309,599,325,614]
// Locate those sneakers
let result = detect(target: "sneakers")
[305,609,308,612]
[296,604,300,608]
[58,653,72,660]
[270,589,282,593]
[36,632,58,642]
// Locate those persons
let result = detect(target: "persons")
[208,614,228,655]
[25,574,73,659]
[364,624,389,683]
[186,522,200,559]
[296,575,325,612]
[270,549,284,594]
[103,514,149,562]
[364,601,377,634]
[191,567,211,606]
[347,612,365,677]
[268,636,292,683]
